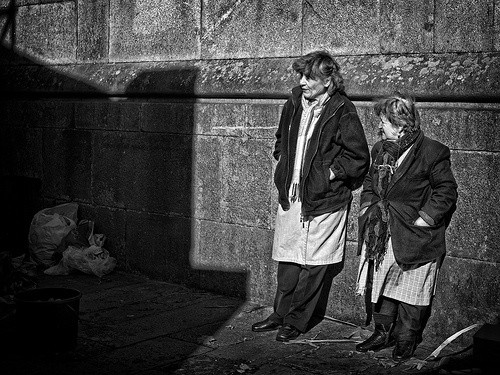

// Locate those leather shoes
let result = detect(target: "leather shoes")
[276,323,301,342]
[252,316,283,331]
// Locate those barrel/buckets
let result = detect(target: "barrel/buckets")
[17,287,81,352]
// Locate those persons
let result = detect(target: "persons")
[356,96,457,362]
[250,51,370,341]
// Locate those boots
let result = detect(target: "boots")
[356,323,396,353]
[392,341,418,361]
